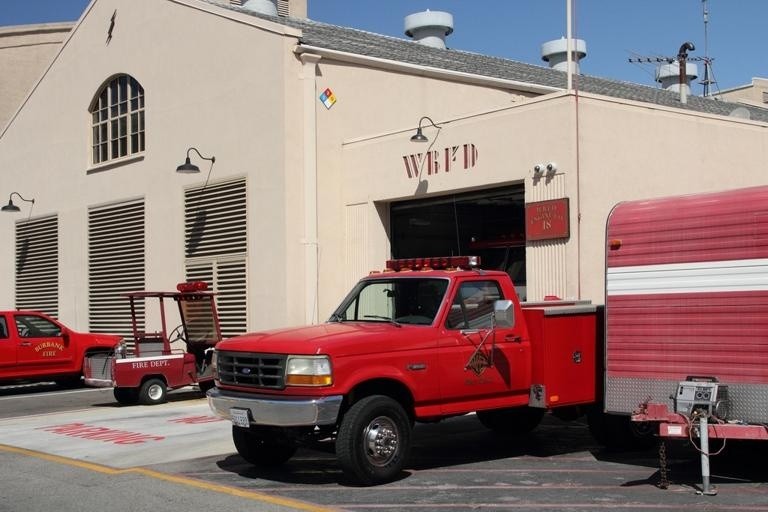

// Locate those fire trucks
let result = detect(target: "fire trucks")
[452,234,526,321]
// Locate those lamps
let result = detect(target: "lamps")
[410,117,441,142]
[176,148,215,173]
[1,192,34,212]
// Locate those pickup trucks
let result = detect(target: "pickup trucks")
[206,256,607,486]
[0,311,128,392]
